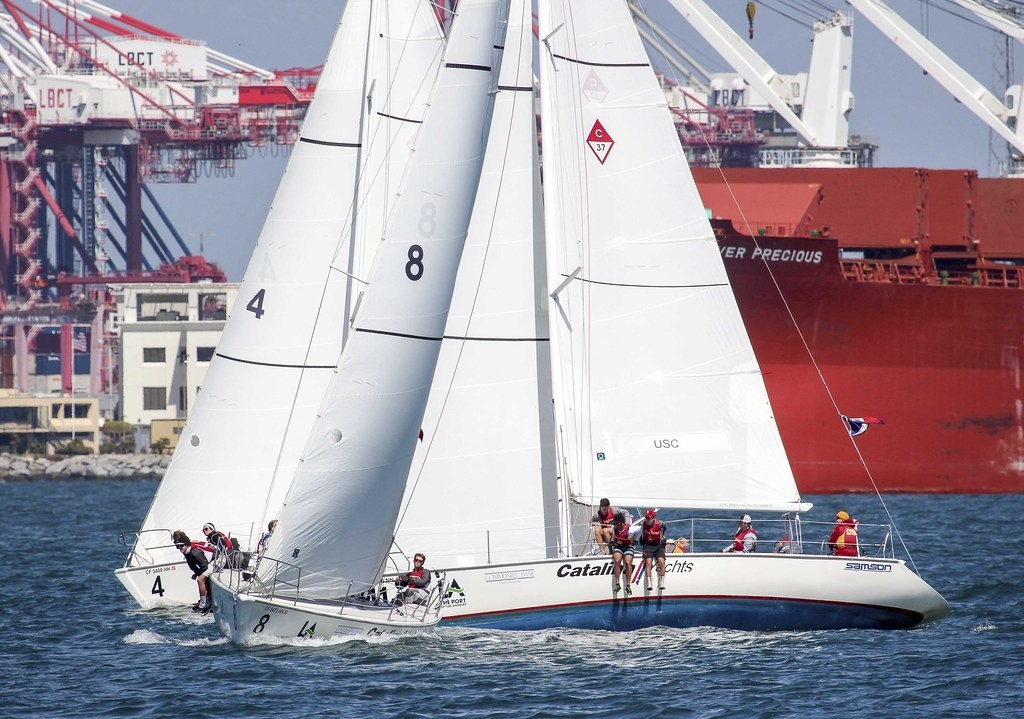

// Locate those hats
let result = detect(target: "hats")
[644,510,655,517]
[835,511,850,520]
[736,514,752,524]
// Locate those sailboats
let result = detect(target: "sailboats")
[114,1,951,631]
[208,0,512,644]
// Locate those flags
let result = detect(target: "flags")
[841,415,884,437]
[73,329,86,352]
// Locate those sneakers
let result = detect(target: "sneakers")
[613,583,621,594]
[625,586,632,595]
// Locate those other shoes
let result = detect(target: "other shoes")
[190,600,213,616]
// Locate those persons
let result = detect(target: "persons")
[198,522,228,609]
[257,520,278,553]
[642,510,667,590]
[590,497,629,555]
[608,512,642,595]
[773,538,802,554]
[721,514,757,553]
[665,537,689,553]
[392,553,432,606]
[203,297,226,320]
[174,536,212,616]
[827,511,858,557]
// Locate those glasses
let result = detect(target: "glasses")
[415,559,423,563]
[177,544,185,549]
[836,517,841,519]
[203,528,209,532]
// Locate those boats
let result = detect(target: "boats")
[628,0,1024,496]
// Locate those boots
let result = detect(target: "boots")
[659,576,665,590]
[646,576,653,590]
